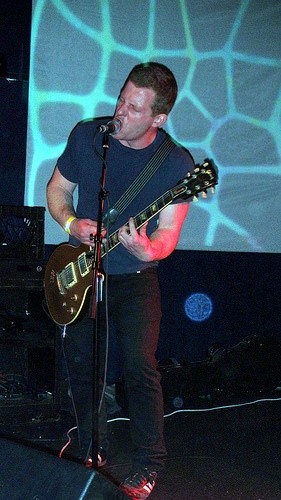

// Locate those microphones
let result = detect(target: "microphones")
[96,120,121,135]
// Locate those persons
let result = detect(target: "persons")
[44,60,200,500]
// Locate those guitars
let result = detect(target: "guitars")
[42,155,220,326]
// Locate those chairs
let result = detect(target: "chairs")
[0,202,63,394]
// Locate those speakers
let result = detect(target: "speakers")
[1,204,68,419]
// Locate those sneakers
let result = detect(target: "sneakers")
[119,469,158,500]
[77,448,110,471]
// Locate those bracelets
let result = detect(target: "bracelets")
[64,216,78,238]
